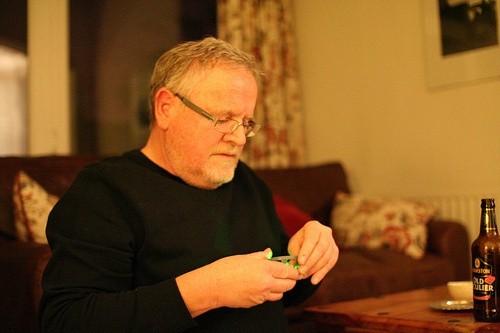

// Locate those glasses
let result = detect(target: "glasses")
[169,89,261,138]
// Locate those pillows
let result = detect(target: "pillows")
[331,190,437,258]
[272,191,312,239]
[13,170,62,243]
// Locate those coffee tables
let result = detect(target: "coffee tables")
[303,285,500,333]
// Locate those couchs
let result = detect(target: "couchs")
[0,155,468,333]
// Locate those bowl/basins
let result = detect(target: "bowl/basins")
[448,282,473,302]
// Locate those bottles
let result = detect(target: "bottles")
[471,199,500,323]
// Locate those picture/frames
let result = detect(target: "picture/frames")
[418,1,500,90]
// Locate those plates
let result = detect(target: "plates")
[428,301,474,310]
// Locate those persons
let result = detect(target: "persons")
[38,36,339,333]
